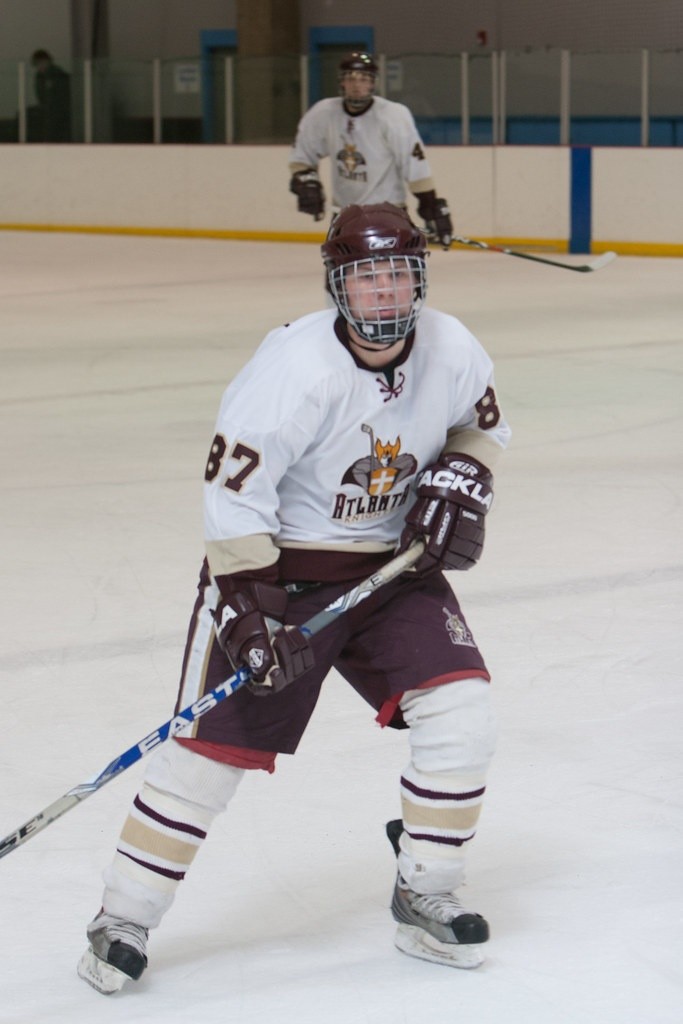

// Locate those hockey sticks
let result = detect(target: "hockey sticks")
[324,203,619,275]
[1,539,428,862]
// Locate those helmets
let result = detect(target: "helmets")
[338,51,378,104]
[321,202,430,344]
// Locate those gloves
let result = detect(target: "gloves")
[414,189,454,245]
[209,563,316,697]
[393,453,494,578]
[291,168,325,221]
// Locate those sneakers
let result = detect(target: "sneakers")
[77,907,149,994]
[386,819,489,968]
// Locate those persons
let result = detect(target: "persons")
[30,49,71,144]
[86,202,511,982]
[287,51,454,251]
